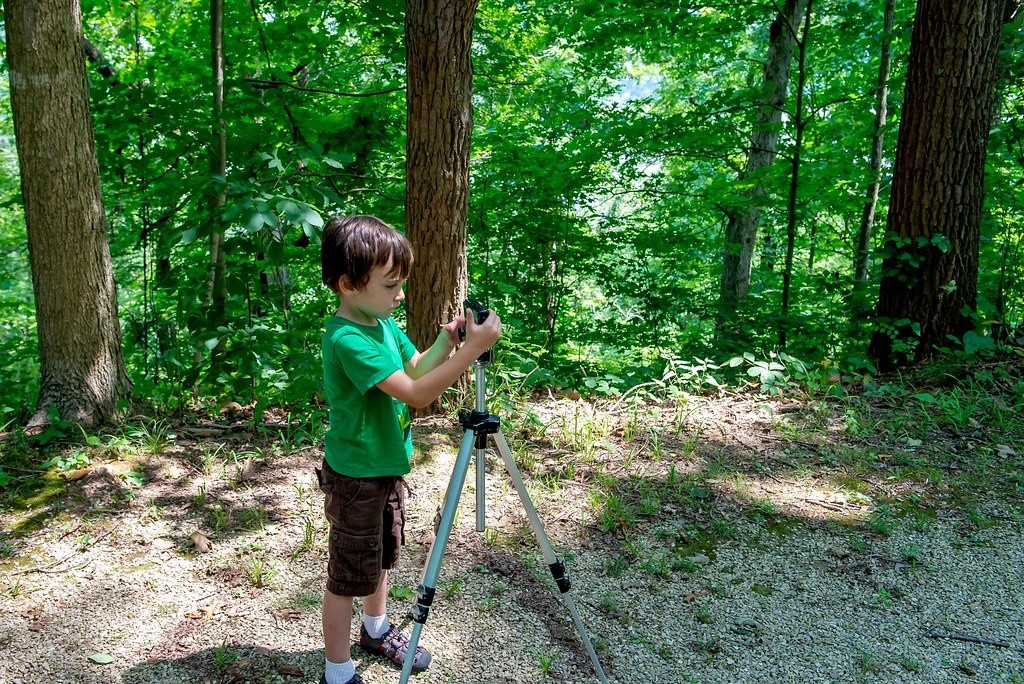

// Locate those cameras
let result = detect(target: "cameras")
[458,299,490,342]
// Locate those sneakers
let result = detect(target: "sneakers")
[360,619,432,670]
[319,670,370,684]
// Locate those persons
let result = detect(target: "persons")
[315,215,503,684]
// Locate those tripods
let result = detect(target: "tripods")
[398,345,608,684]
